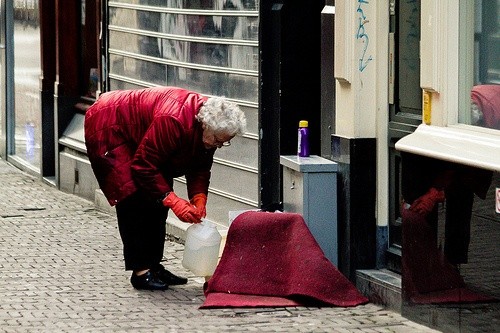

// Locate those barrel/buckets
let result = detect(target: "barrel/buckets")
[182,220,222,277]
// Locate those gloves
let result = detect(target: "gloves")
[161,192,207,223]
[399,188,443,229]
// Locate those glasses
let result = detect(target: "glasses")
[214,134,231,147]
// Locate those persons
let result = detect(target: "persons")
[84,86,246,291]
[398,151,495,303]
[470,83,500,130]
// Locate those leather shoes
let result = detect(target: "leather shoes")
[152,265,188,285]
[130,271,168,290]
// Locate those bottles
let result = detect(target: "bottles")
[298,120,310,156]
